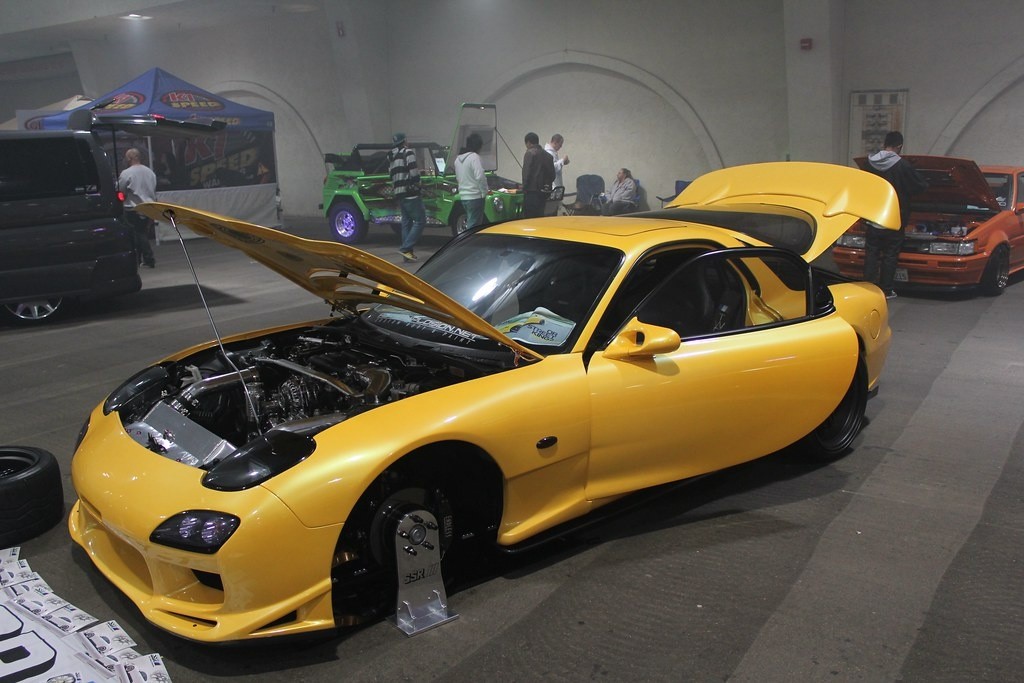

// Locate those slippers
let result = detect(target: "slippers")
[398,251,418,260]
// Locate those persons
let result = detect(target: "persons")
[544,134,570,215]
[602,168,638,216]
[454,133,489,229]
[861,131,928,299]
[522,133,555,218]
[386,132,425,261]
[116,148,158,268]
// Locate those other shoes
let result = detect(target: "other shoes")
[140,263,154,268]
[886,292,897,299]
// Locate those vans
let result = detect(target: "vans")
[0,110,227,326]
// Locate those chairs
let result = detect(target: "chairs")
[601,178,640,206]
[561,174,605,216]
[656,180,692,209]
[619,254,715,339]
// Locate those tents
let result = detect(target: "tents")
[43,67,282,241]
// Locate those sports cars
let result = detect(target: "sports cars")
[65,161,902,639]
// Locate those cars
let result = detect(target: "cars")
[319,103,565,245]
[834,154,1024,298]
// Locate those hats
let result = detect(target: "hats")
[392,133,407,145]
